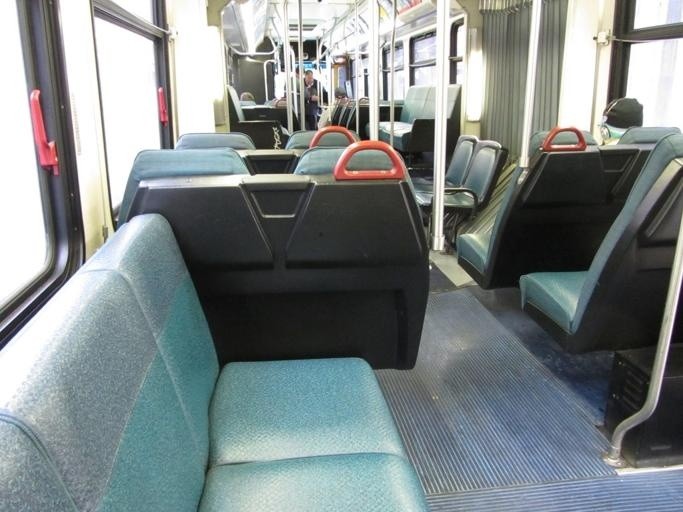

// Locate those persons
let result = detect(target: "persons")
[284,66,326,130]
[600,98,643,145]
[335,87,353,107]
[240,92,254,101]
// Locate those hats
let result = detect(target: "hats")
[333,86,345,100]
[598,95,644,140]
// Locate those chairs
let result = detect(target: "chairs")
[0,86,682,511]
